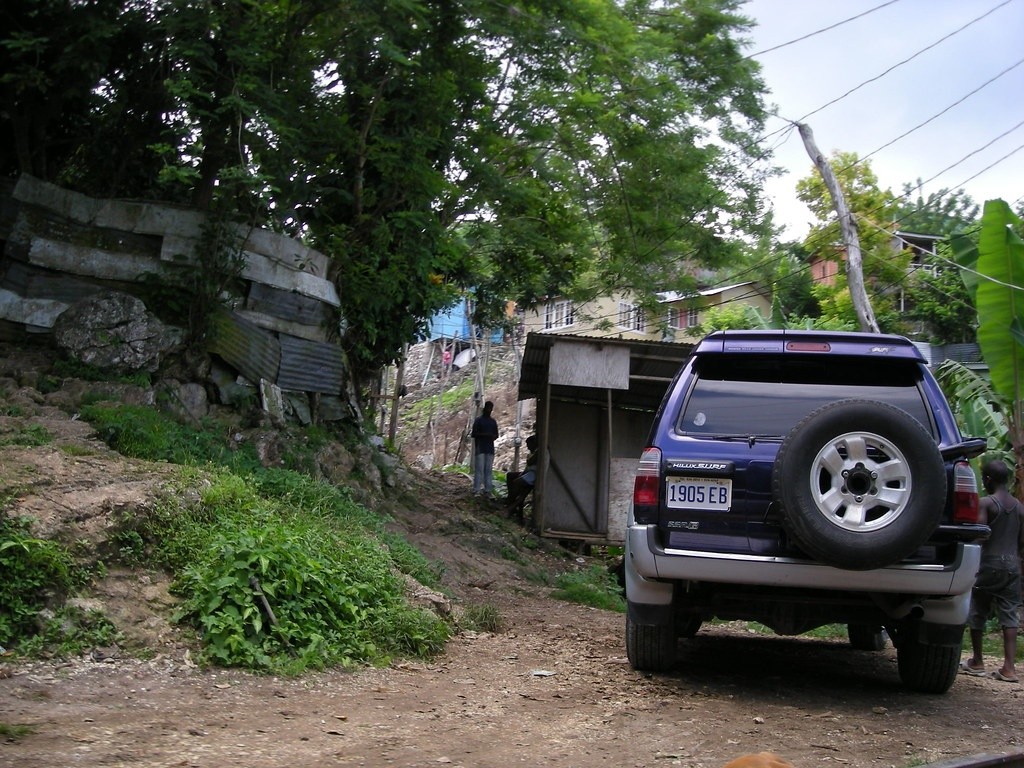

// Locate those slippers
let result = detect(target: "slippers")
[957,659,985,677]
[987,668,1019,681]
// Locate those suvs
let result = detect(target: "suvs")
[623,328,990,694]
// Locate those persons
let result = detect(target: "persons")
[498,423,537,518]
[956,461,1024,682]
[471,401,498,499]
